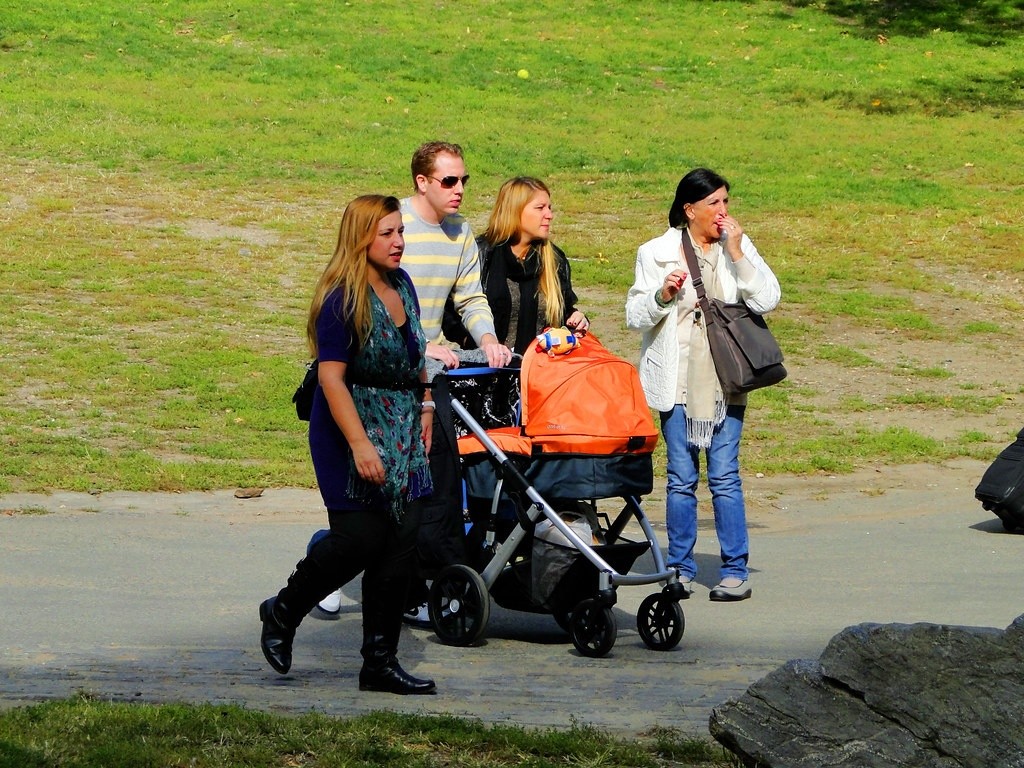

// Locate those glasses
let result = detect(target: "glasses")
[426,174,469,189]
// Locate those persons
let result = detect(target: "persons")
[306,141,512,623]
[259,195,436,695]
[440,176,591,439]
[625,168,781,600]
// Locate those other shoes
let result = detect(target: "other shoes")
[709,577,752,602]
[662,575,693,599]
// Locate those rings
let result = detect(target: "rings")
[731,225,736,230]
[500,353,506,356]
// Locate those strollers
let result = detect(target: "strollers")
[428,325,690,658]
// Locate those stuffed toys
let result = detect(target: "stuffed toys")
[536,326,581,358]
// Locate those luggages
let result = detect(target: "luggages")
[976,429,1024,532]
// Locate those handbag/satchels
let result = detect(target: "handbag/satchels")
[292,359,321,420]
[704,299,788,397]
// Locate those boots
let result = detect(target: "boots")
[359,579,436,692]
[259,557,327,675]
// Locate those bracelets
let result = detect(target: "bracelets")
[421,400,436,411]
[656,288,675,307]
